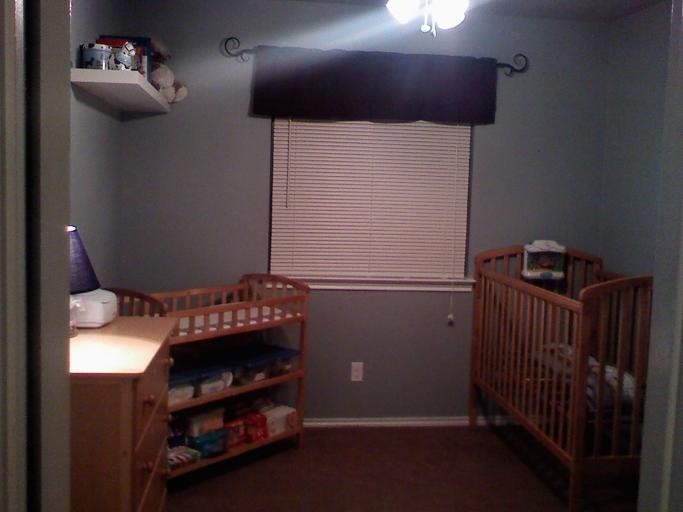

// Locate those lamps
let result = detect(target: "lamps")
[386,0,469,37]
[67,225,101,337]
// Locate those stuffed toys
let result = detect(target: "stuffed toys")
[151,64,187,103]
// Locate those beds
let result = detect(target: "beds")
[468,243,653,511]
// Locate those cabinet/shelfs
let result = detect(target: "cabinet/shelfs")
[104,273,311,482]
[69,316,175,511]
